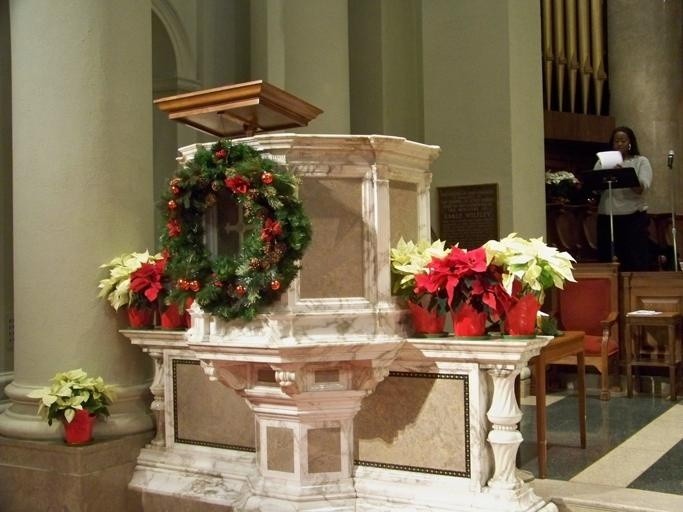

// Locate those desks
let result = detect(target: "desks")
[514,331,587,478]
[625,309,683,400]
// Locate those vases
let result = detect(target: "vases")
[60,409,96,445]
[158,302,186,330]
[127,304,153,329]
[406,298,445,334]
[452,300,489,337]
[499,279,540,336]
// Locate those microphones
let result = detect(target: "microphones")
[668,149,675,170]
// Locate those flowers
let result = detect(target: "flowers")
[27,368,119,431]
[96,248,164,310]
[414,243,521,311]
[130,258,187,302]
[390,235,467,308]
[482,232,578,298]
[160,140,311,319]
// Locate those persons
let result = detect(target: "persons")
[593,126,653,271]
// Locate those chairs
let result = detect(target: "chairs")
[531,262,620,401]
